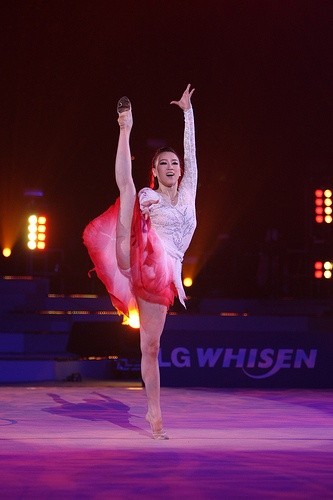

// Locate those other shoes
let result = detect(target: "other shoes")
[116,96,132,126]
[146,413,170,440]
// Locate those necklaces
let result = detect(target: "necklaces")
[159,189,177,203]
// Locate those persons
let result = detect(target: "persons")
[81,83,199,441]
